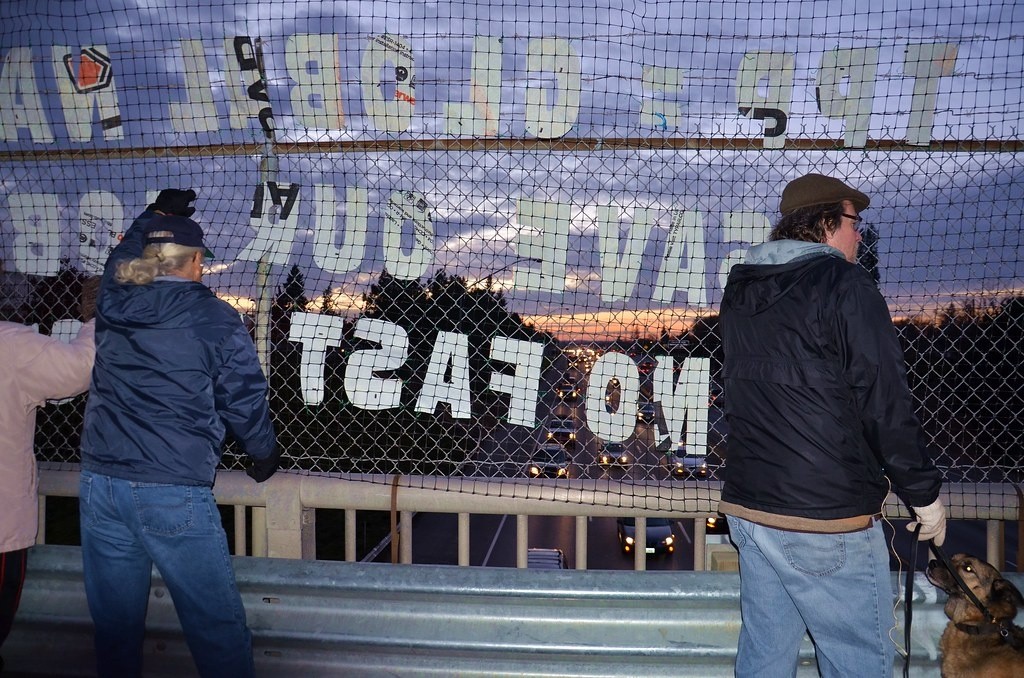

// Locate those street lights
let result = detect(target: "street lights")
[466,258,543,291]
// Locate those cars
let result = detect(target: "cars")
[667,446,708,476]
[616,515,675,559]
[526,548,570,570]
[530,443,572,479]
[678,440,686,447]
[598,441,629,469]
[637,401,655,424]
[606,388,612,411]
[706,518,729,534]
[547,415,575,449]
[555,377,581,401]
[561,346,683,375]
[708,389,722,408]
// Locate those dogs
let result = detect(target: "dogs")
[925,552,1024,678]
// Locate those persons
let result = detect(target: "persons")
[0,275,102,647]
[79,188,280,678]
[718,174,946,677]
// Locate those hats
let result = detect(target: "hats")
[141,217,217,259]
[779,174,871,211]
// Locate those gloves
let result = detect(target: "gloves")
[905,499,948,546]
[157,188,196,217]
